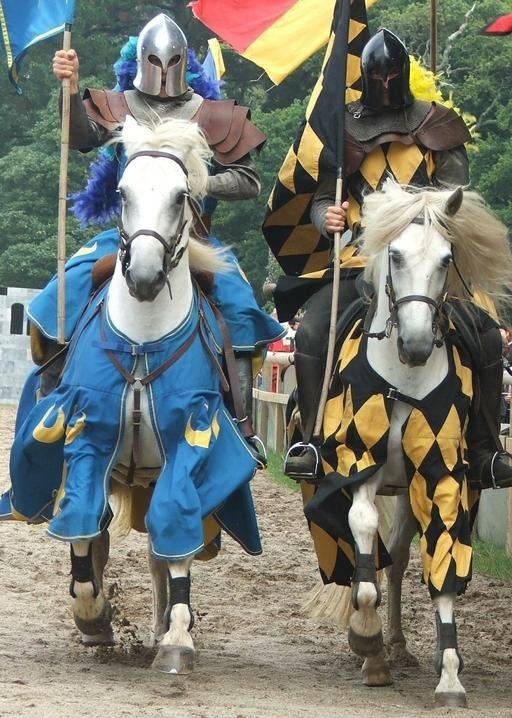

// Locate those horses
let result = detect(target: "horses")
[49,114,236,675]
[286,179,512,709]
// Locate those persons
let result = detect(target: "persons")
[284,25,511,490]
[26,9,273,471]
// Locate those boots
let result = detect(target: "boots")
[285,351,327,484]
[467,360,511,488]
[38,338,63,396]
[225,356,268,469]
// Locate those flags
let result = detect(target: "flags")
[191,0,379,87]
[0,1,78,91]
[261,0,371,279]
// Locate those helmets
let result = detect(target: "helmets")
[361,29,414,110]
[133,12,188,98]
